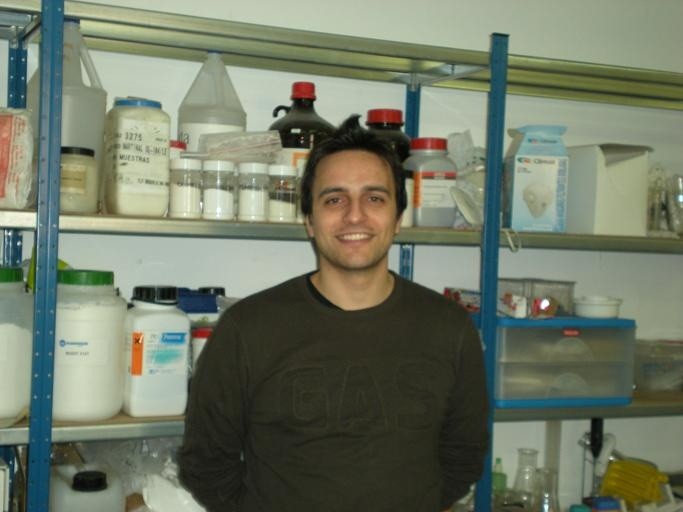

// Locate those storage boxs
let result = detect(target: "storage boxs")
[469,313,637,408]
[567,144,649,237]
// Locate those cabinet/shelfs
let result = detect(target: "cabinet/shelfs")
[0,0,683,512]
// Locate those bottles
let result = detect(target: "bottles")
[166,158,298,222]
[514,445,557,512]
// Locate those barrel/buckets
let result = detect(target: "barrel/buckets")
[46,443,127,510]
[177,50,244,151]
[24,20,107,212]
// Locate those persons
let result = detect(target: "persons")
[173,130,492,511]
